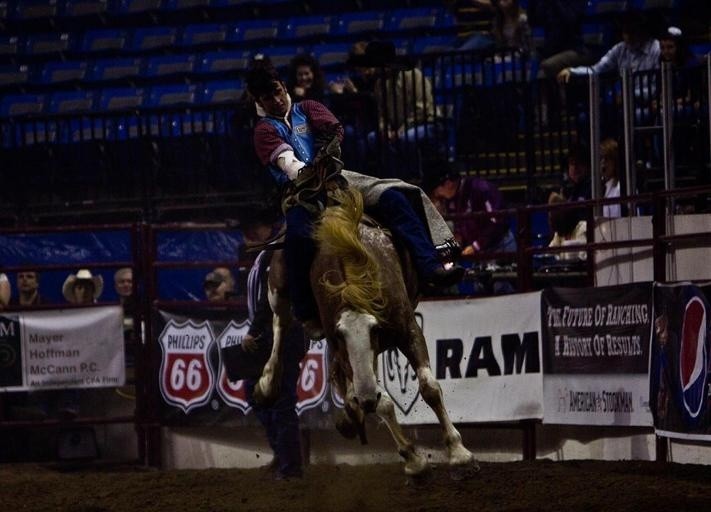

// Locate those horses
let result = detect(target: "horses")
[253,187,481,489]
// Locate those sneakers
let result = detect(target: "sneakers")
[31,403,48,420]
[57,402,80,418]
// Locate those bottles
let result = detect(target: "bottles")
[652,304,674,430]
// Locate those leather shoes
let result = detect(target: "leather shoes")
[301,320,324,341]
[419,268,464,296]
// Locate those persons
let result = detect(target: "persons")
[1,0,710,480]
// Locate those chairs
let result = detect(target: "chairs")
[0,1,539,149]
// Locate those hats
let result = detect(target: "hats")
[61,268,103,304]
[201,272,225,284]
[201,280,224,287]
